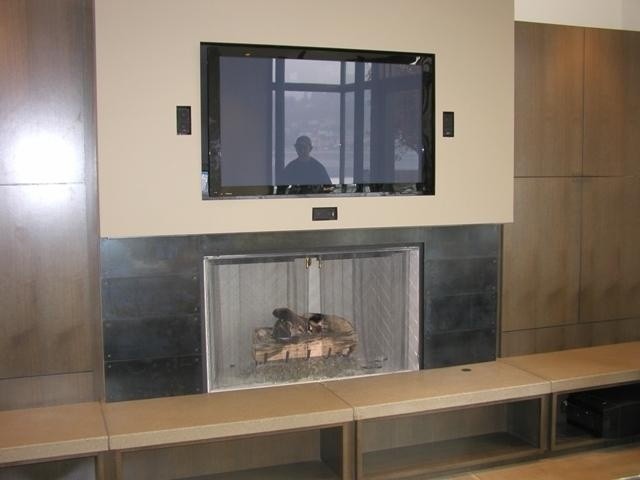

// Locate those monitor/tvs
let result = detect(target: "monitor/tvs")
[209,44,432,197]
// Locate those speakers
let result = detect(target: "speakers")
[443,112,454,136]
[313,208,337,220]
[177,106,191,135]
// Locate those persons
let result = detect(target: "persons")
[284,136,334,184]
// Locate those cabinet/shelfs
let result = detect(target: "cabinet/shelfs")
[0,341,640,480]
